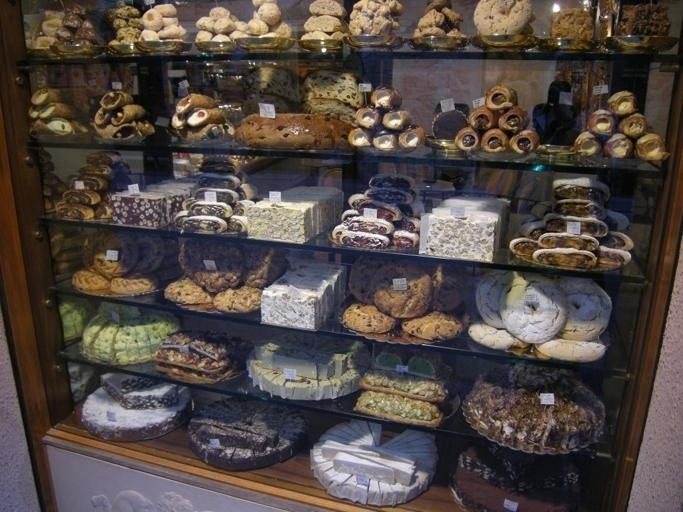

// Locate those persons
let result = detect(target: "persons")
[531,79,570,140]
[540,104,581,147]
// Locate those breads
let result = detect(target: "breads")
[27,0,671,512]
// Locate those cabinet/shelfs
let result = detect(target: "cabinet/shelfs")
[1,0,683,511]
